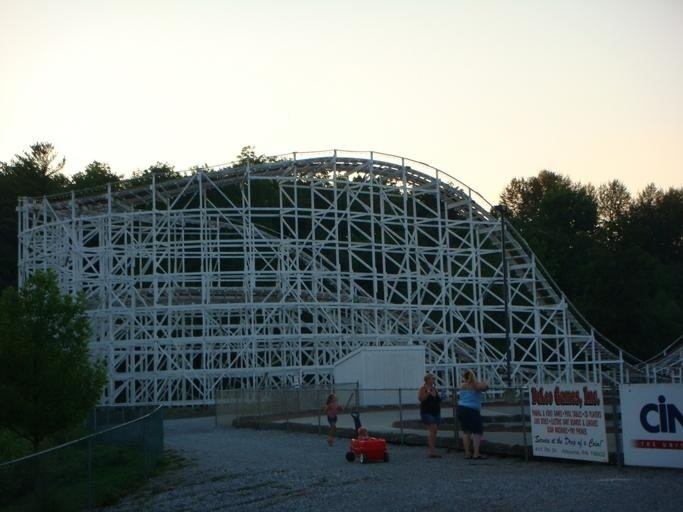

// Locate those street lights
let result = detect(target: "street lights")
[489,202,514,387]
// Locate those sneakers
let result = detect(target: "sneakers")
[430,454,441,458]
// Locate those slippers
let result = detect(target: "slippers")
[464,456,472,459]
[473,456,487,459]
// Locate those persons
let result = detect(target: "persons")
[320,393,344,448]
[417,372,442,458]
[455,370,488,459]
[357,427,370,441]
